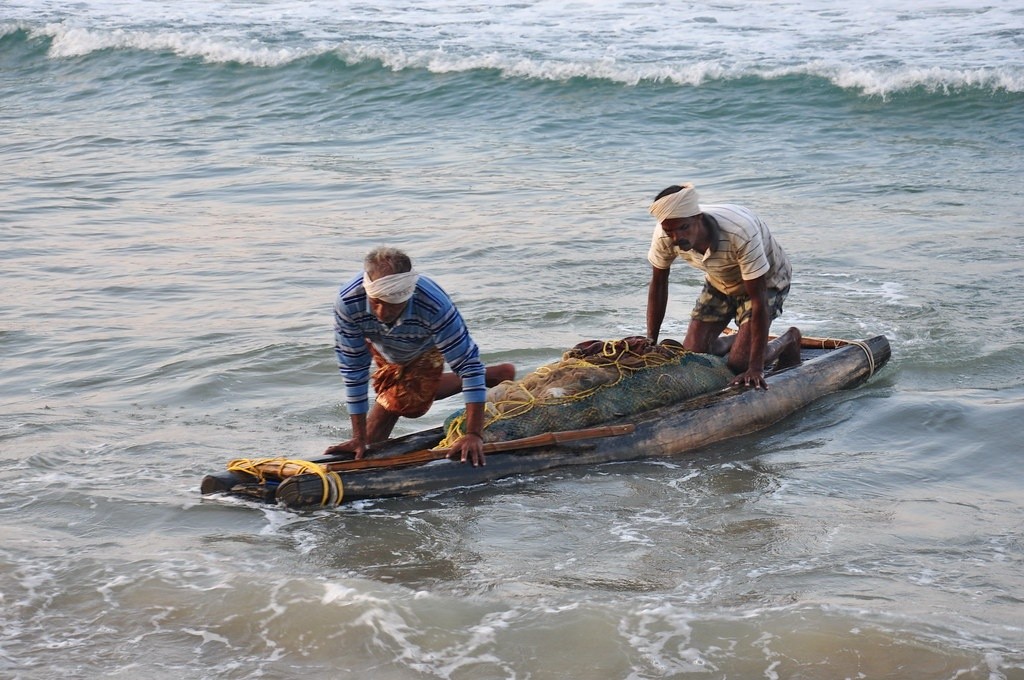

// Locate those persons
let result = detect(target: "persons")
[323,246,516,466]
[647,182,801,390]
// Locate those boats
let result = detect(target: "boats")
[200,327,891,510]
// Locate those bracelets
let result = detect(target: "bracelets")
[465,431,484,441]
